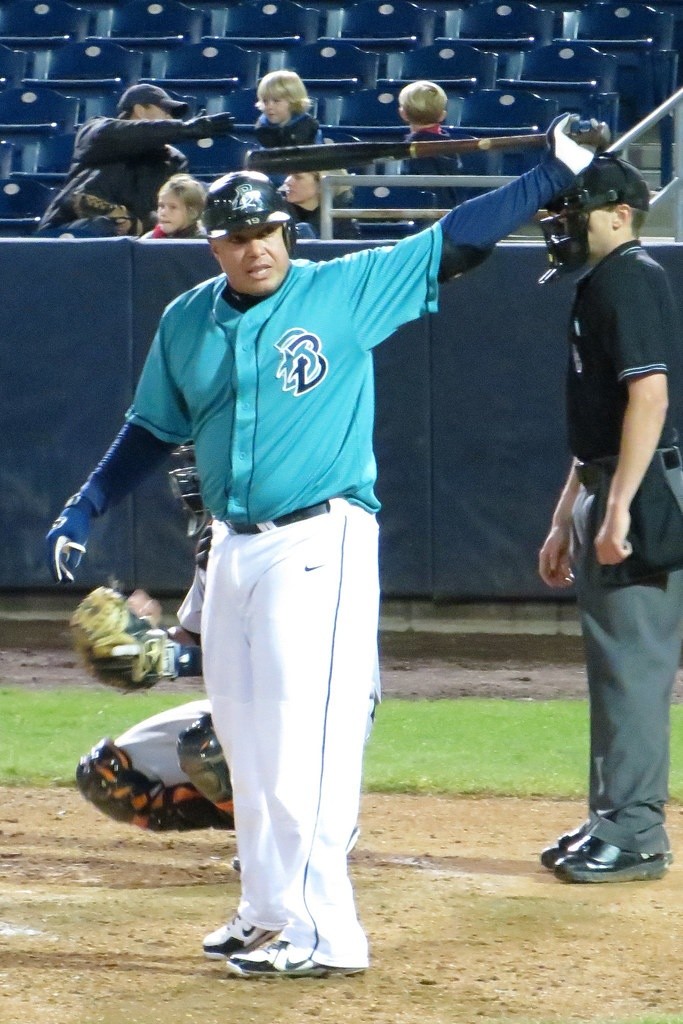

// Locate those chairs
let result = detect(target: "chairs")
[0,0,683,240]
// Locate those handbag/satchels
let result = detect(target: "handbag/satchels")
[590,453,682,582]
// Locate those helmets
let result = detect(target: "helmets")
[200,169,297,254]
[168,439,212,538]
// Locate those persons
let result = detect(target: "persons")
[254,69,325,146]
[398,80,463,177]
[137,174,209,239]
[537,158,683,883]
[71,443,383,874]
[284,138,360,240]
[43,112,598,975]
[36,83,237,237]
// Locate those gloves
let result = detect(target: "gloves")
[179,107,236,141]
[545,110,611,178]
[45,490,98,585]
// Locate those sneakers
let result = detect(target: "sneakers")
[201,914,283,959]
[222,932,365,979]
[538,827,667,884]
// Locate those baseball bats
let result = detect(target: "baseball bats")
[244,120,613,176]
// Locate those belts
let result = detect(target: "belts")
[222,498,330,535]
[574,448,681,484]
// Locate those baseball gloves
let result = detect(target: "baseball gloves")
[65,584,168,694]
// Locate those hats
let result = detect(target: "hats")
[117,83,189,120]
[542,157,651,215]
